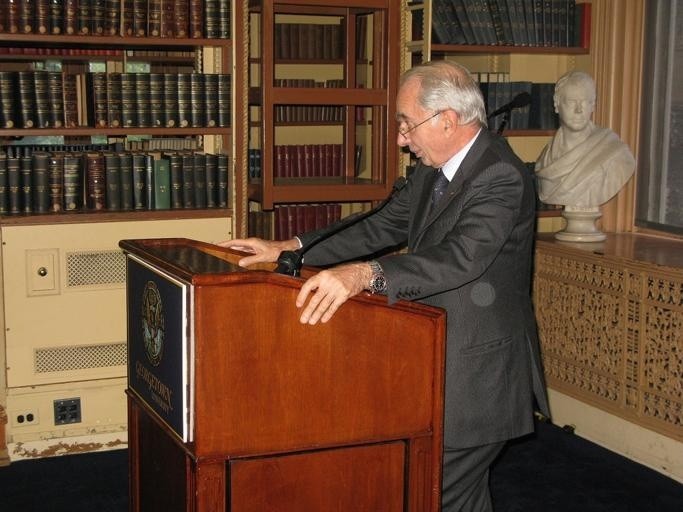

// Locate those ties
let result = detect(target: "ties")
[431,170,447,212]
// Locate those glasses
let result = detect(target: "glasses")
[399,112,443,139]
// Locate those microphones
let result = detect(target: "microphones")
[488,92,531,119]
[274,175,407,276]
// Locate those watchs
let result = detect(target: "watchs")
[368,261,387,294]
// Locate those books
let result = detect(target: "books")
[410,0,595,130]
[1,136,229,214]
[0,43,228,128]
[248,23,372,242]
[0,1,229,39]
[524,163,563,210]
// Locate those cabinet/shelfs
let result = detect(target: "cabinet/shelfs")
[233,0,400,245]
[531,229,681,487]
[395,1,591,218]
[0,1,238,225]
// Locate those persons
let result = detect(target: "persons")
[217,59,551,512]
[534,71,635,211]
